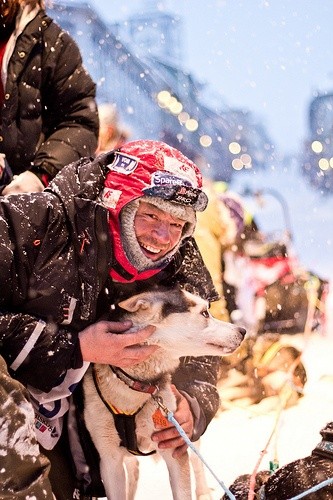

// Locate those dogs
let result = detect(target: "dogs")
[81,289,248,500]
[224,332,307,415]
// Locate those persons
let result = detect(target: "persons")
[0,0,101,198]
[0,104,333,499]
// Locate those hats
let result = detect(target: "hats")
[103,139,203,283]
